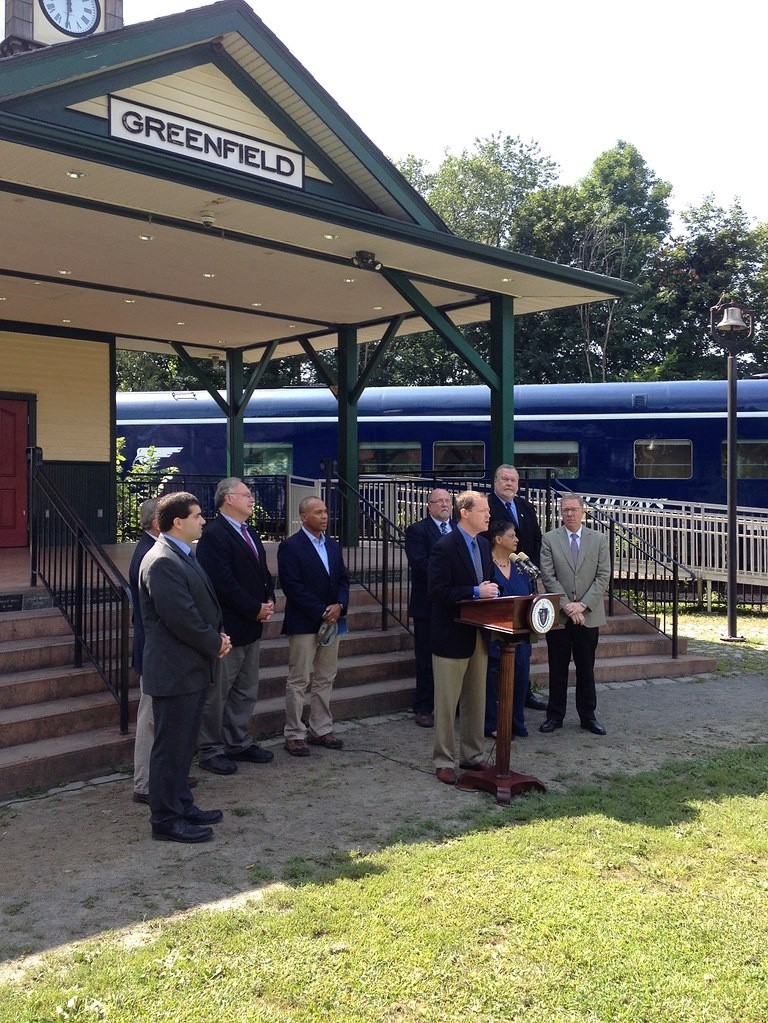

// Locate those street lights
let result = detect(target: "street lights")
[710,291,754,641]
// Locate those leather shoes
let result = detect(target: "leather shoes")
[459,760,492,771]
[151,819,214,842]
[540,718,563,732]
[436,768,456,784]
[179,806,223,824]
[581,718,606,735]
[524,689,548,710]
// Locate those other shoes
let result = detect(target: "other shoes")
[186,776,197,788]
[485,728,528,741]
[133,792,150,805]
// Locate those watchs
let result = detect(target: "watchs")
[581,601,587,608]
[340,602,343,609]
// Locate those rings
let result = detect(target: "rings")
[332,619,334,621]
[570,608,572,610]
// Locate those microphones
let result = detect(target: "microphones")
[508,552,542,577]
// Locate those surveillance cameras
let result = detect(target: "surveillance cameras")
[200,215,216,227]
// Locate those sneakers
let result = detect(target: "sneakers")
[306,730,342,749]
[199,754,237,775]
[415,711,434,727]
[224,745,274,763]
[285,738,310,756]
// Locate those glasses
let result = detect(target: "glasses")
[430,499,451,505]
[561,508,582,513]
[224,492,255,498]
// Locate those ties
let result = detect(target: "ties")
[441,523,447,535]
[471,538,482,585]
[571,534,578,567]
[241,523,259,562]
[506,502,519,531]
[189,550,208,584]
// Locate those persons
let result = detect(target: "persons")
[485,465,547,709]
[139,493,234,841]
[132,499,199,800]
[426,490,505,783]
[539,494,612,734]
[276,495,349,756]
[485,515,532,740]
[404,487,460,727]
[195,475,276,774]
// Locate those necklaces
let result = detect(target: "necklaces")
[493,556,509,567]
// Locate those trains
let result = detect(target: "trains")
[116,379,768,587]
